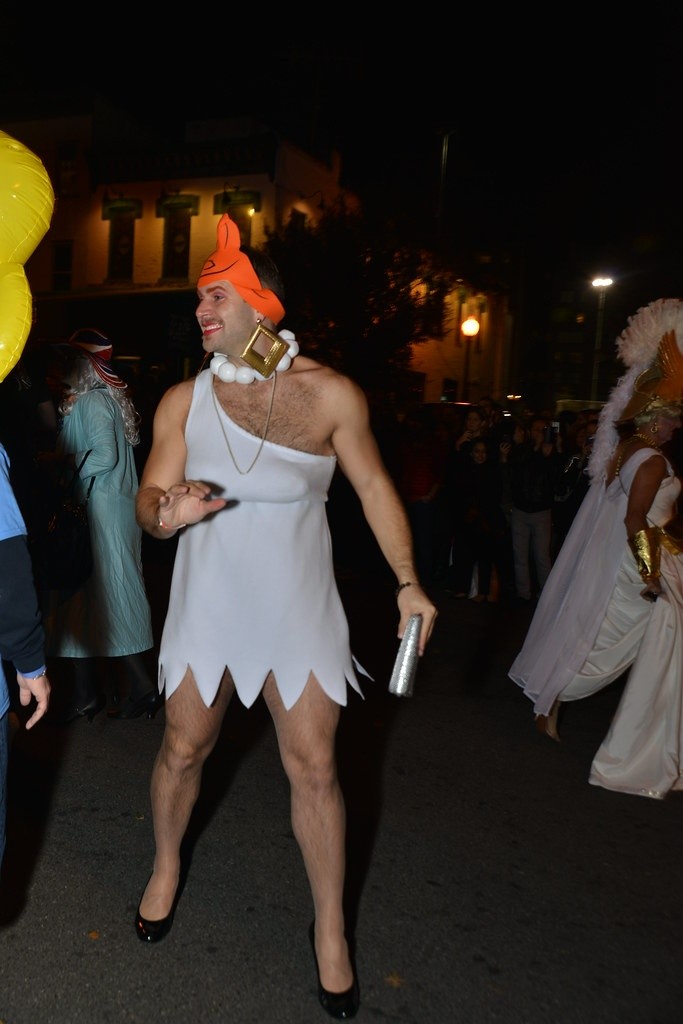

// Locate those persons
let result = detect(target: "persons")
[510,297,683,798]
[374,391,599,602]
[136,248,439,1020]
[0,325,160,727]
[0,441,51,869]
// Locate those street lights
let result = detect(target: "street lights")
[586,268,620,402]
[460,317,480,401]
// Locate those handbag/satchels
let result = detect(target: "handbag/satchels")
[31,450,96,590]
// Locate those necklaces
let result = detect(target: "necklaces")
[209,330,300,384]
[632,433,662,453]
[210,370,276,474]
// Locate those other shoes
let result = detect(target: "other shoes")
[473,594,486,601]
[453,592,466,598]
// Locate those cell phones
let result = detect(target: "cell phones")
[587,435,595,444]
[542,426,558,443]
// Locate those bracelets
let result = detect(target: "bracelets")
[397,582,410,595]
[32,670,46,680]
[157,516,187,529]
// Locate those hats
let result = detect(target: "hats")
[53,324,128,390]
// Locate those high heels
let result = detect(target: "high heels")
[308,919,361,1019]
[107,689,164,721]
[42,694,104,729]
[136,866,184,944]
